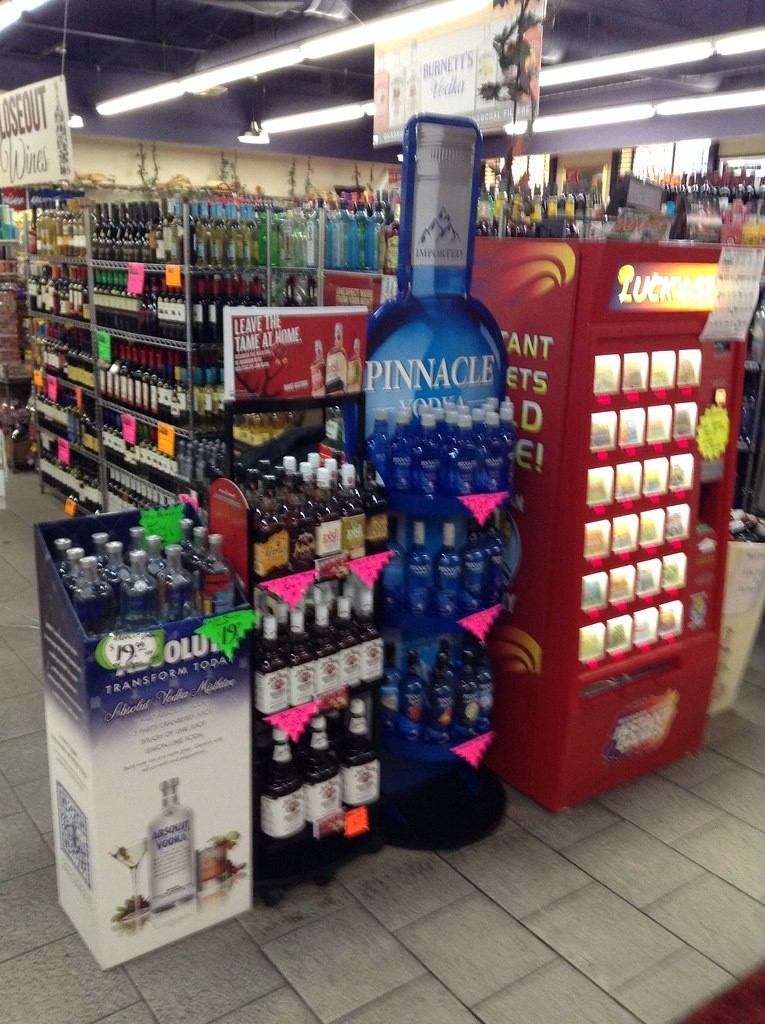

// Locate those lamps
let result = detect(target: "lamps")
[52,0,82,129]
[94,0,765,146]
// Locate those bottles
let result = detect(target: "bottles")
[47,511,237,636]
[22,196,403,532]
[341,112,505,848]
[664,164,765,245]
[232,401,515,859]
[469,177,604,238]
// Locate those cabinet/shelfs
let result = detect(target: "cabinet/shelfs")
[0,201,519,909]
